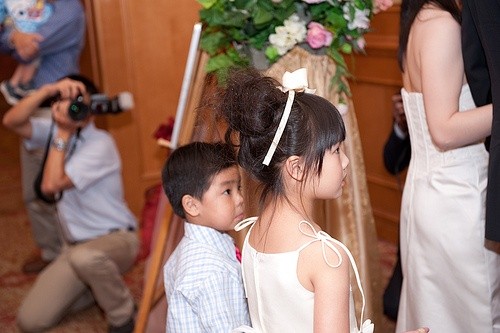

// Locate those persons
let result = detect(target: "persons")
[0,0,85,273]
[397,0,500,333]
[0,0,53,105]
[162,141,251,333]
[1,73,139,333]
[222,69,366,333]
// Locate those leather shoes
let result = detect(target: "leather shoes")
[108,302,138,333]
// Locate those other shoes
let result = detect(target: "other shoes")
[22,260,48,273]
[0,80,36,105]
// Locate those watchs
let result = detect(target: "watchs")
[51,139,65,151]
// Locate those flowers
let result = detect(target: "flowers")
[198,0,394,103]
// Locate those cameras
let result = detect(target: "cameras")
[69,92,134,121]
